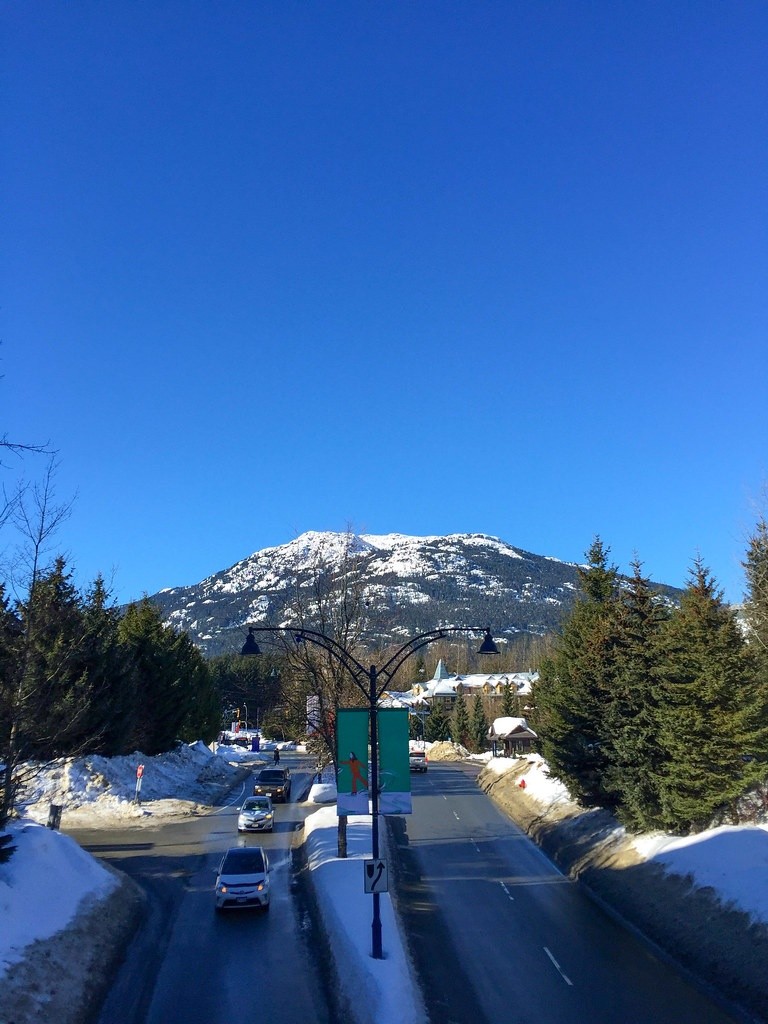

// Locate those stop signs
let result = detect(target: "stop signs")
[137,766,144,778]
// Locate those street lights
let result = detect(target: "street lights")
[237,627,501,958]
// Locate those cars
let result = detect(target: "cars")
[214,846,275,914]
[236,796,276,833]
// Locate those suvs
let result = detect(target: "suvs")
[253,764,292,803]
[408,752,427,772]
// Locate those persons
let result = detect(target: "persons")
[274,748,280,765]
